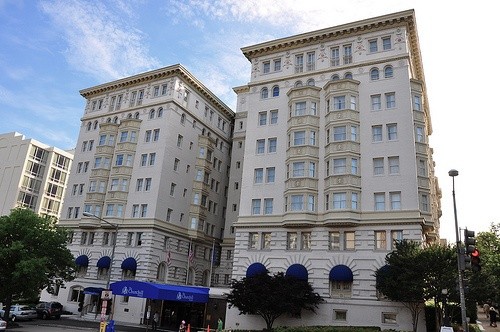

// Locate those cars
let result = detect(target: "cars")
[0,317,7,331]
[0,305,38,322]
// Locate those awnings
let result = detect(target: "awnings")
[120,256,137,270]
[96,256,110,267]
[75,254,89,267]
[329,264,353,283]
[286,264,308,280]
[83,287,105,295]
[246,262,267,277]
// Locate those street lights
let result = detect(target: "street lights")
[83,211,119,332]
[448,168,469,332]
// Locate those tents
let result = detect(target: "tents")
[152,312,159,331]
[109,280,209,332]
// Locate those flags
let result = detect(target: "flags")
[209,243,220,266]
[167,245,170,264]
[187,240,194,265]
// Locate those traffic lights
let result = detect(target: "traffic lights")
[464,229,475,256]
[471,248,481,272]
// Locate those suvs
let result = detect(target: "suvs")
[31,301,63,320]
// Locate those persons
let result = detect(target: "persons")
[178,320,187,332]
[217,317,223,332]
[483,303,499,327]
